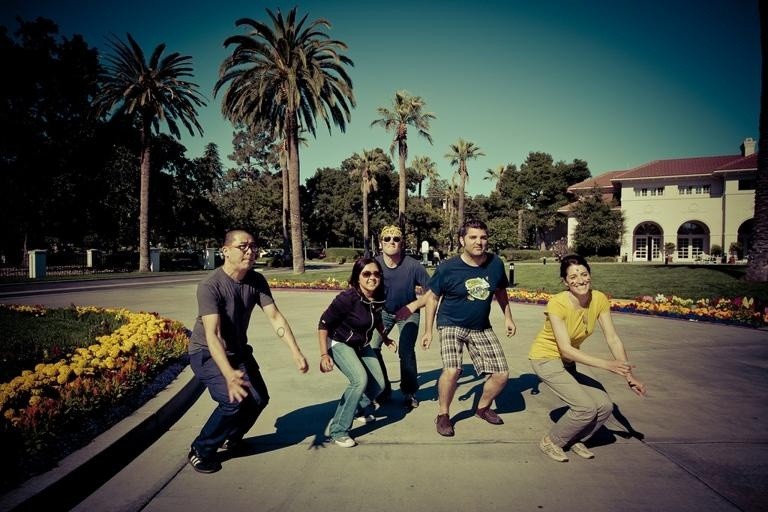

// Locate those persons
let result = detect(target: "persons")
[527,255,649,463]
[419,219,518,438]
[432,247,443,267]
[188,229,310,474]
[372,225,432,411]
[316,257,398,447]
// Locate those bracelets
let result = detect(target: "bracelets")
[320,353,327,357]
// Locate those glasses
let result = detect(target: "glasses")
[362,270,382,277]
[383,236,402,243]
[227,244,257,253]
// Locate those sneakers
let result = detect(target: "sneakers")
[221,438,256,454]
[475,407,504,425]
[539,435,568,462]
[355,414,375,422]
[406,393,420,408]
[330,432,357,447]
[433,414,455,437]
[570,441,594,460]
[188,445,217,473]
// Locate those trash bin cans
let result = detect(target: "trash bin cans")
[28,249,47,278]
[149,247,161,272]
[86,248,98,268]
[203,248,215,270]
